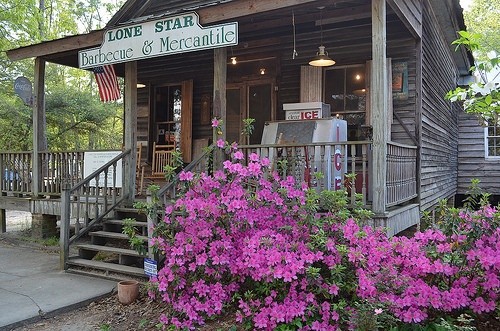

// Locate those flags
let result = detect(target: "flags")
[92,64,121,102]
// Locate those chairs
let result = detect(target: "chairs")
[139,142,178,196]
[266,132,296,179]
[119,141,142,195]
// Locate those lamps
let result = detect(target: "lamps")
[309,6,336,67]
